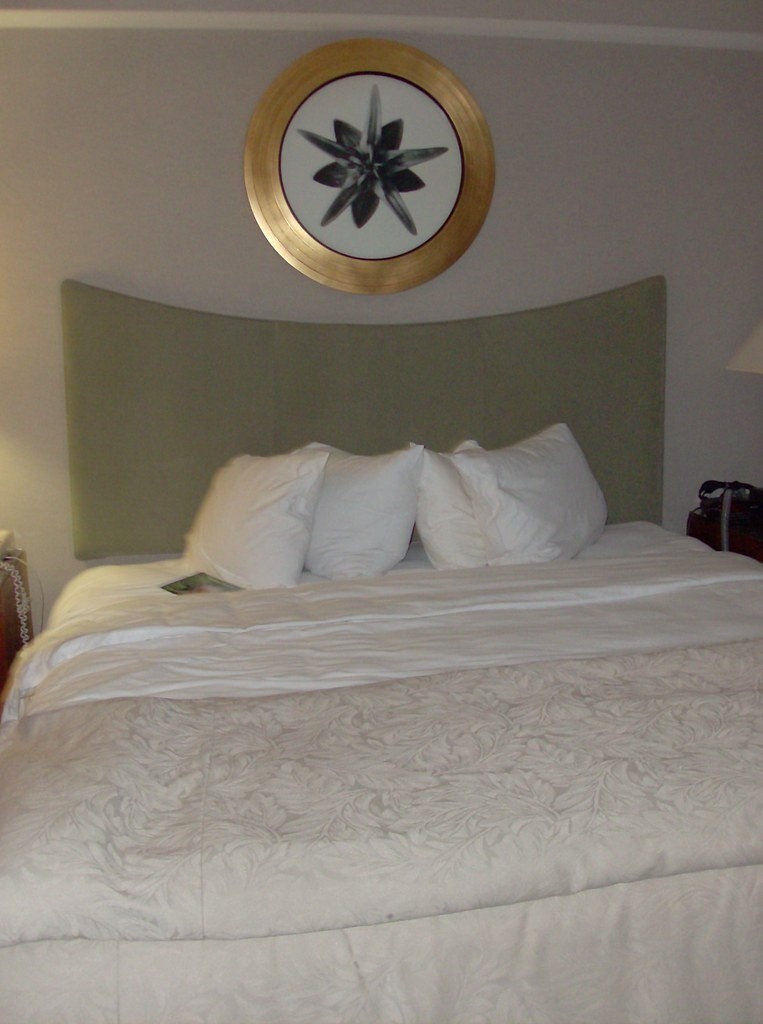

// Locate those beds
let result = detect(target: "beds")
[3,272,763,1022]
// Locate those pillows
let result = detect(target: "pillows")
[440,419,608,568]
[178,445,330,593]
[407,436,495,573]
[302,440,425,583]
